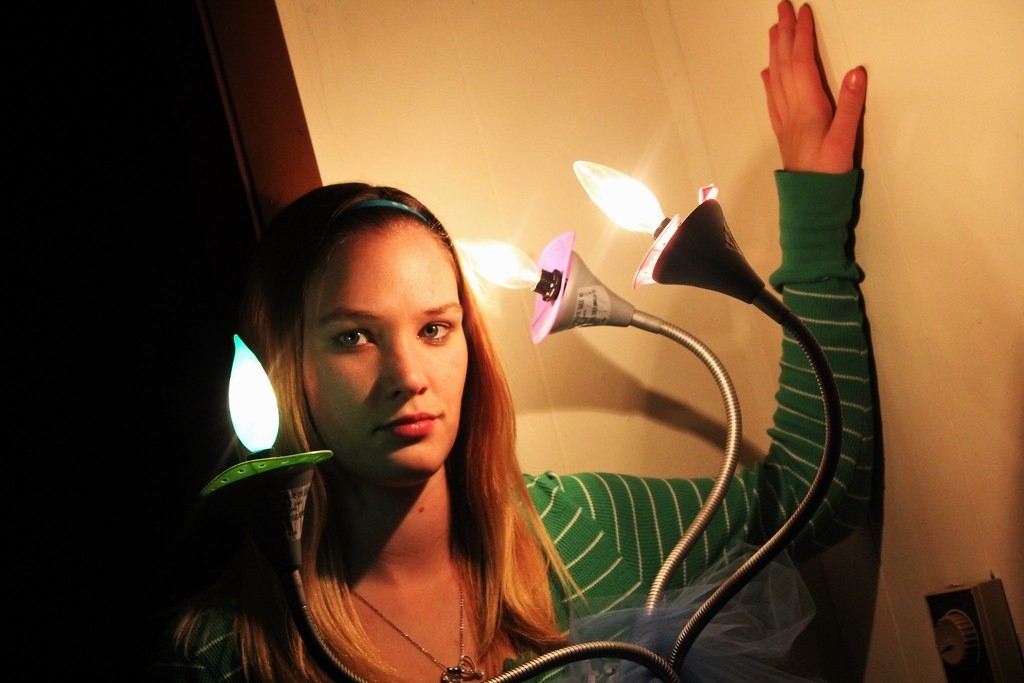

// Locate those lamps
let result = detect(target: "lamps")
[571,159,842,672]
[449,236,742,618]
[199,329,677,683]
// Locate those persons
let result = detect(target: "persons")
[159,2,883,681]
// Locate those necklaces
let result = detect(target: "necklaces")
[347,573,485,681]
